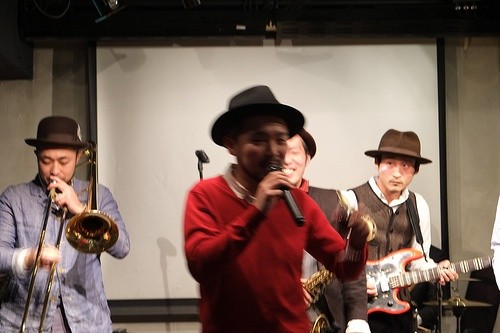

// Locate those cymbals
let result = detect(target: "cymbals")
[422,296,492,309]
[456,278,481,282]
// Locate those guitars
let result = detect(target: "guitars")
[366,247,494,314]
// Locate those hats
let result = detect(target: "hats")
[298,128,317,159]
[364,129,433,164]
[25,116,89,149]
[211,86,304,146]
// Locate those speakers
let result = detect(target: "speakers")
[419,299,493,333]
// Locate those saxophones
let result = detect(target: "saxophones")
[303,197,378,333]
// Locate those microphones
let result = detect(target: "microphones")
[264,151,304,227]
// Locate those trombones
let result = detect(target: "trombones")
[20,139,119,333]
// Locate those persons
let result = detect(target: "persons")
[340,129,459,333]
[0,116,130,333]
[183,86,369,333]
[281,127,371,333]
[490,196,500,290]
